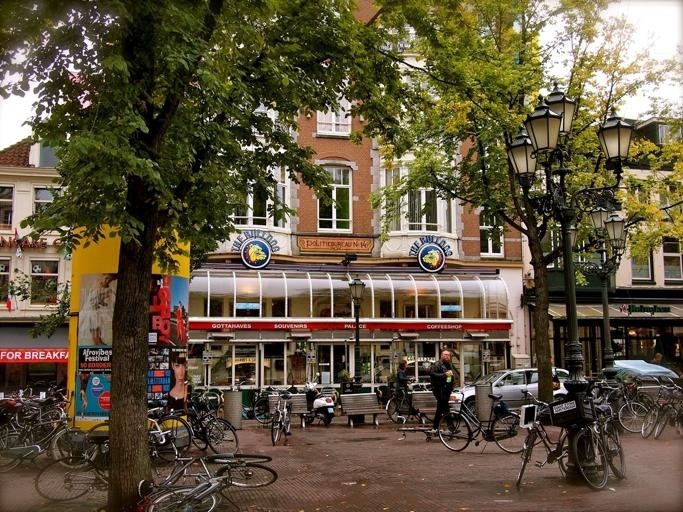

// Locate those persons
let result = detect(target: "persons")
[394,360,415,421]
[651,353,663,365]
[85,272,117,346]
[175,301,185,342]
[168,357,184,410]
[77,374,91,408]
[429,350,461,434]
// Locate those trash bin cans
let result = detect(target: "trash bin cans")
[224,392,243,431]
[475,382,493,422]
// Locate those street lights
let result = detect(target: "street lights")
[559,207,627,433]
[347,274,366,383]
[506,81,634,486]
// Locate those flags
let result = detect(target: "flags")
[14,229,22,258]
[6,283,15,312]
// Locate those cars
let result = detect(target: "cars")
[448,367,569,417]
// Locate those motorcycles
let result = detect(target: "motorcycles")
[300,372,334,425]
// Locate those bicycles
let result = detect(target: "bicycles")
[397,416,477,442]
[0,385,97,472]
[617,376,683,439]
[517,386,625,490]
[386,379,421,424]
[438,384,529,454]
[35,389,278,512]
[254,395,292,446]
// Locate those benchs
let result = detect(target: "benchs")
[266,390,457,429]
